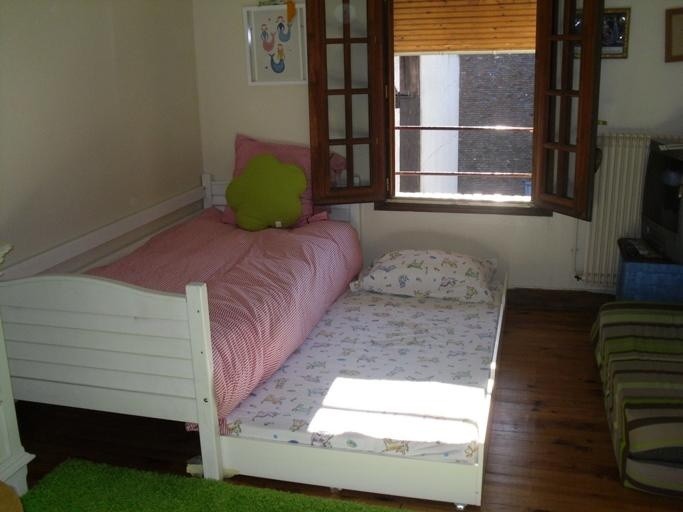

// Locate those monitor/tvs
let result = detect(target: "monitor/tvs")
[641,140,683,257]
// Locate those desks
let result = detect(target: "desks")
[617,238,683,301]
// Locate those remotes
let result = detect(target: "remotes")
[628,239,648,255]
[625,243,637,257]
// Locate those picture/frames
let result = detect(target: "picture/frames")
[574,8,630,59]
[243,3,308,87]
[665,7,683,62]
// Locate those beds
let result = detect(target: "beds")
[220,267,508,508]
[0,173,363,480]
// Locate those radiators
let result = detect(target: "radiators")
[571,132,683,288]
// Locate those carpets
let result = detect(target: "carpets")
[21,458,403,512]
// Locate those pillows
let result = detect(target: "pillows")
[225,155,307,232]
[350,250,498,305]
[222,135,346,227]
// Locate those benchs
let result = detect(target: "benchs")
[590,301,683,498]
